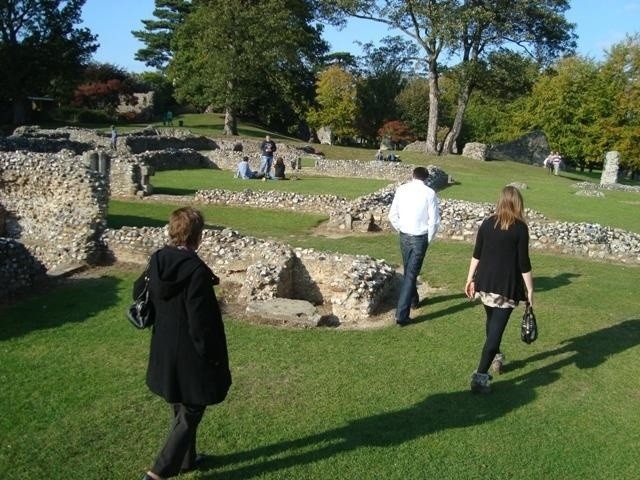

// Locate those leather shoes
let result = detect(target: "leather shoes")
[181,454,204,473]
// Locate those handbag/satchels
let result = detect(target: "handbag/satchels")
[520,312,538,344]
[126,288,155,330]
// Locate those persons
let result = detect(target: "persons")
[384,152,401,162]
[260,134,276,179]
[388,168,440,326]
[376,150,383,160]
[145,109,172,126]
[109,125,118,152]
[464,185,533,394]
[196,107,210,116]
[274,158,285,180]
[548,151,555,175]
[552,151,562,176]
[134,208,233,479]
[235,156,266,180]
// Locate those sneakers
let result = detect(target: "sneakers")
[395,293,419,325]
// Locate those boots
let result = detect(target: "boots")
[491,352,505,375]
[471,371,493,395]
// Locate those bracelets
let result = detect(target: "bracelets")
[465,279,476,283]
